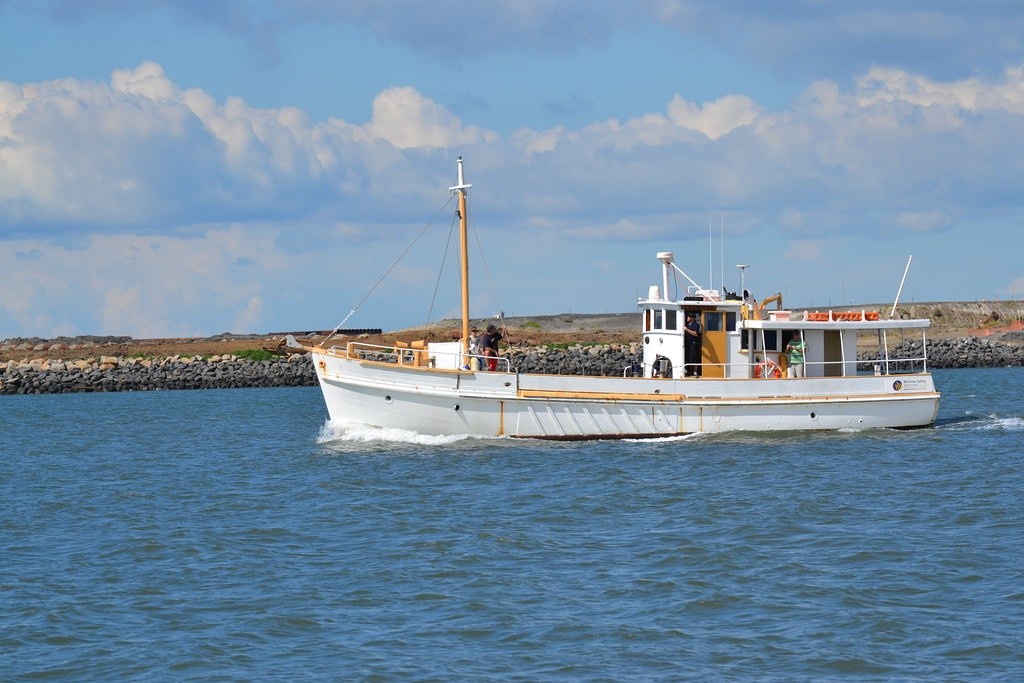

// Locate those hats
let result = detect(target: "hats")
[687,313,696,318]
[487,324,495,330]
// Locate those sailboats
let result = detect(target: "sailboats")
[260,153,942,441]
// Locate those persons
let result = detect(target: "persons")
[468,326,484,371]
[785,330,807,378]
[482,324,506,371]
[684,314,700,376]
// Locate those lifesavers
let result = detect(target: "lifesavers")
[754,360,780,379]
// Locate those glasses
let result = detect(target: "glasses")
[472,330,476,332]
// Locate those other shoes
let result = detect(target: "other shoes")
[685,371,698,376]
[485,367,490,370]
